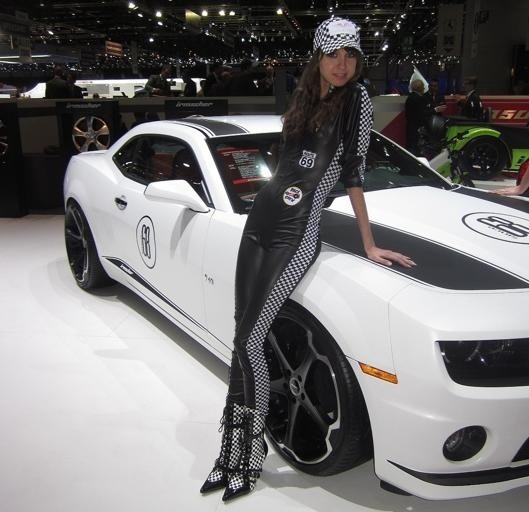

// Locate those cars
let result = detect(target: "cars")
[63,114,527,500]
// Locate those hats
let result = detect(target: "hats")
[313,17,364,56]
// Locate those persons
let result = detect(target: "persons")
[357,76,485,158]
[199,15,417,501]
[45,60,298,99]
[489,158,529,197]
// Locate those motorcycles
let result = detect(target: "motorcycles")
[416,109,514,188]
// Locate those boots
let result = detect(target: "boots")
[222,404,268,501]
[200,402,244,493]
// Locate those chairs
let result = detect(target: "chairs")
[151,152,173,179]
[173,148,201,179]
